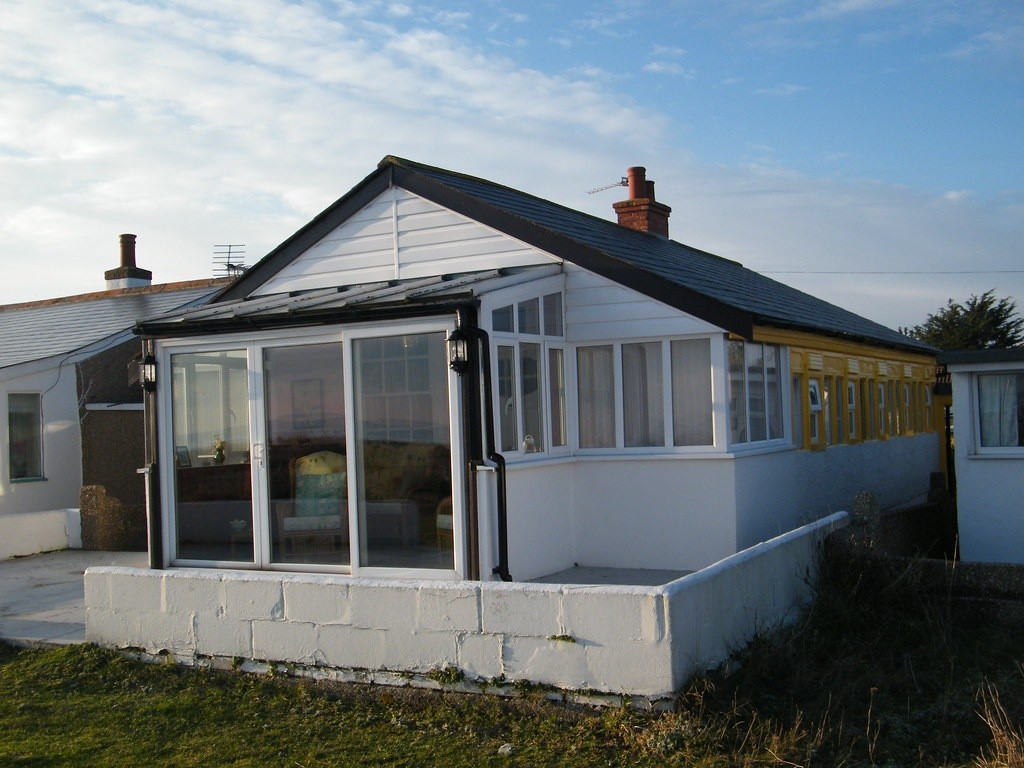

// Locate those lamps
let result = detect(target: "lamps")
[139,352,157,396]
[445,326,471,377]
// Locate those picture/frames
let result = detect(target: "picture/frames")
[176,446,191,467]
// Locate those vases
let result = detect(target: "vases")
[214,448,225,465]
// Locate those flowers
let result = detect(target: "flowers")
[212,435,226,453]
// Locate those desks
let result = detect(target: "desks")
[231,529,252,559]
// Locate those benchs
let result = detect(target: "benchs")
[362,439,445,543]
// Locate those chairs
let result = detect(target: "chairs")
[280,451,350,557]
[436,496,453,551]
